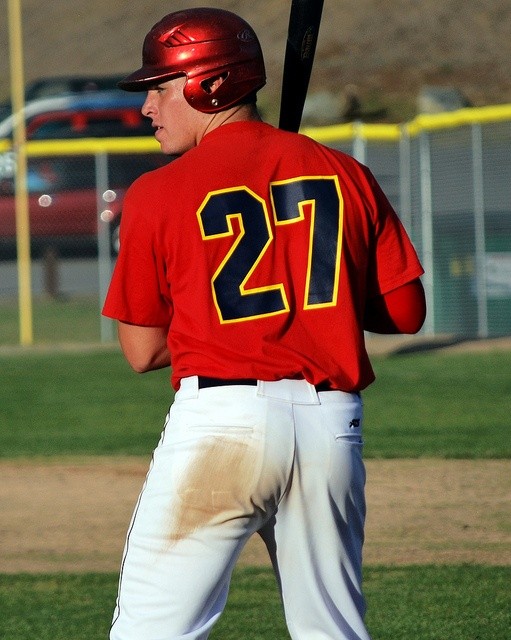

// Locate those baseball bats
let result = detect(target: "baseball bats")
[279,0,324,134]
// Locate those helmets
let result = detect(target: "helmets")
[117,7,266,112]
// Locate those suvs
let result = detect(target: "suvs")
[0,108,186,257]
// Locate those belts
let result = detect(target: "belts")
[198,375,334,393]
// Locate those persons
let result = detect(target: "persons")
[101,7,427,640]
